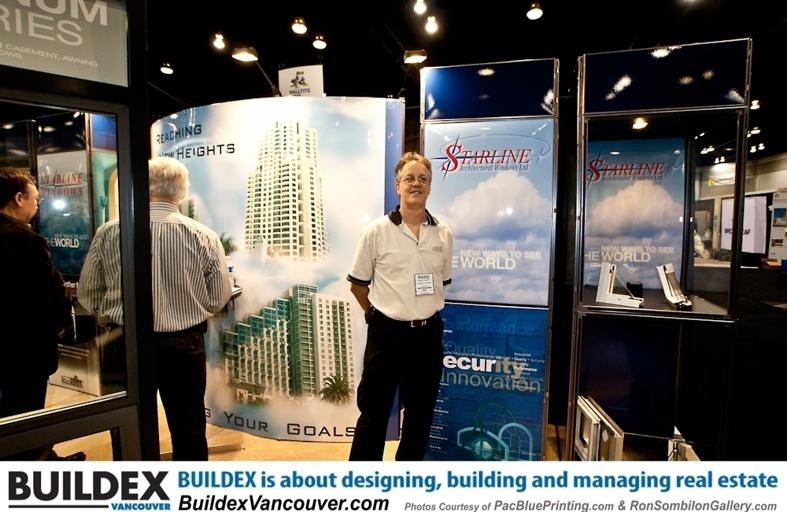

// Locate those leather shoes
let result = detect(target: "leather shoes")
[59,452,85,461]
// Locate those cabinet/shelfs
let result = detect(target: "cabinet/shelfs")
[580,287,737,444]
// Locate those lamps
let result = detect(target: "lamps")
[160,0,544,97]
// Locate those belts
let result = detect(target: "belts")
[391,315,440,329]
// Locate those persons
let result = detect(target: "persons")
[76,156,233,460]
[0,165,88,461]
[349,152,451,462]
